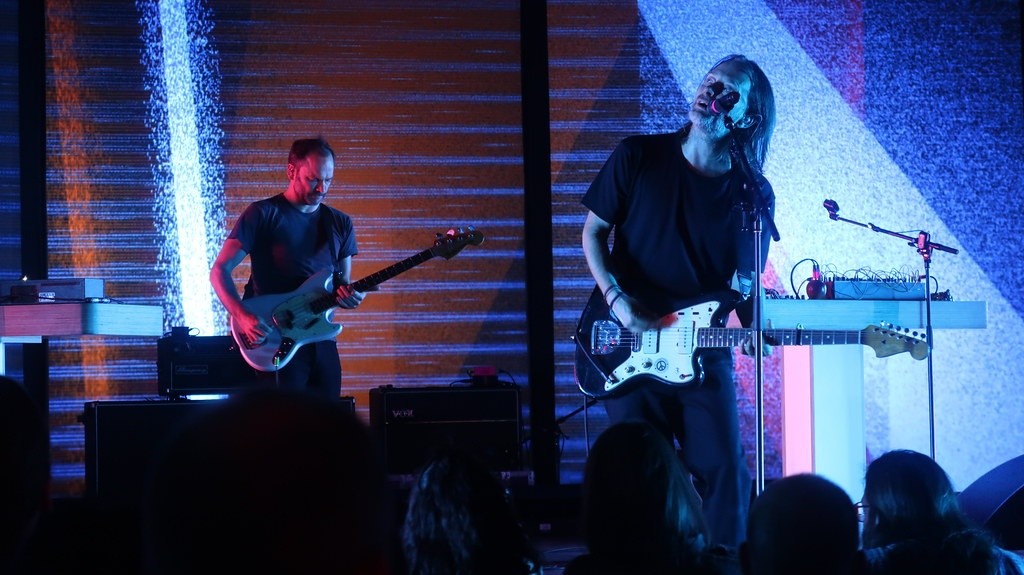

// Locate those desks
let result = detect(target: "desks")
[0,300,165,411]
[753,298,989,523]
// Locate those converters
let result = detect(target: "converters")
[471,366,499,385]
[170,326,189,336]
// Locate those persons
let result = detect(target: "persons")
[1,376,1024,575]
[579,55,782,546]
[208,137,380,426]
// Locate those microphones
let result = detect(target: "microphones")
[707,92,740,116]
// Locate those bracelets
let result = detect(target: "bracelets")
[605,282,625,310]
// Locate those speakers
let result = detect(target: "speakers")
[156,336,258,396]
[369,385,525,478]
[85,397,356,504]
[956,454,1024,552]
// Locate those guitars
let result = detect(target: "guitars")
[569,289,933,402]
[224,221,489,377]
